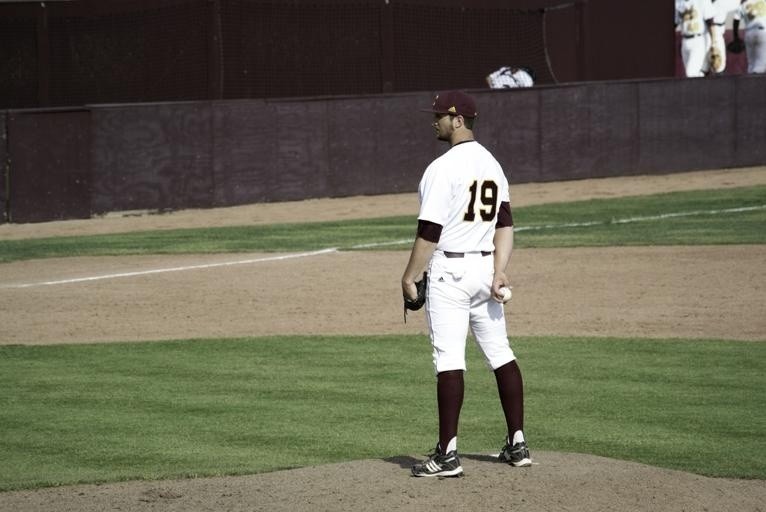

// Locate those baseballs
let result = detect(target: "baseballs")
[497,286,511,302]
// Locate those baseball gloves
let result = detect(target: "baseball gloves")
[403,272,427,311]
[710,45,723,74]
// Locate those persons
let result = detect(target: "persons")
[675,1,716,80]
[401,90,533,479]
[740,0,766,75]
[709,1,742,76]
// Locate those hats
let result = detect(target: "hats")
[421,91,476,118]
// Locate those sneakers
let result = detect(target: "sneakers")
[411,450,462,477]
[498,442,531,467]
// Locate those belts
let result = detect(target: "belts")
[710,22,724,26]
[444,252,491,258]
[681,34,700,38]
